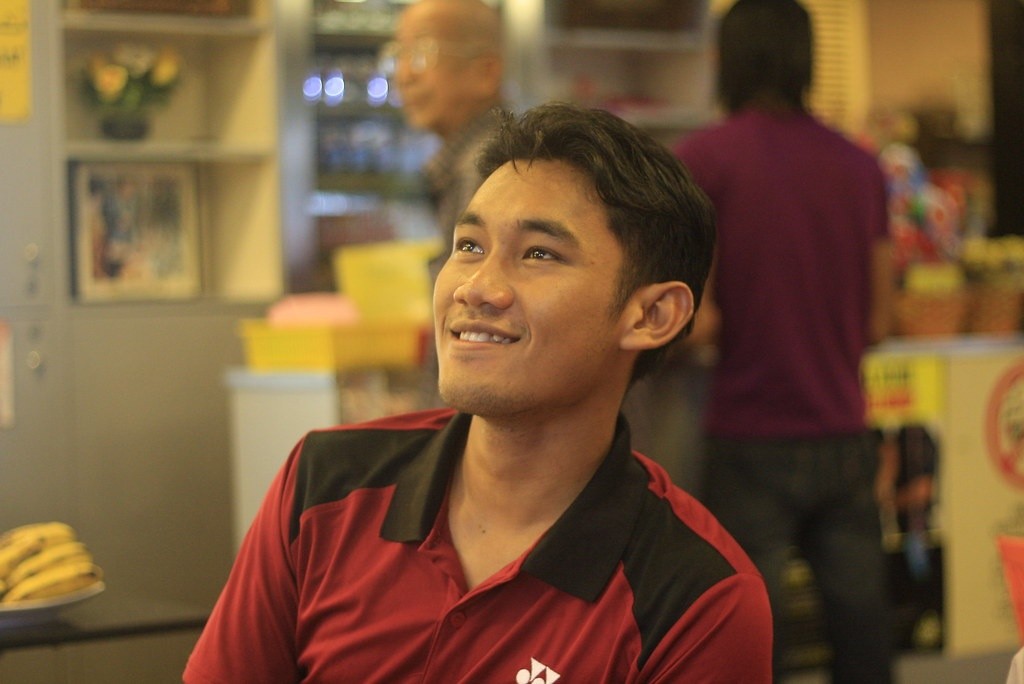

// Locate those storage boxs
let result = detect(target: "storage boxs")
[241,319,422,374]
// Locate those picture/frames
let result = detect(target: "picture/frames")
[67,161,211,307]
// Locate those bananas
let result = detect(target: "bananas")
[0,521,104,604]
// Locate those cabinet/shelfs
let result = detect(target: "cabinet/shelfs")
[60,10,268,166]
[546,0,713,134]
[312,9,419,216]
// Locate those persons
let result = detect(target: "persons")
[386,0,896,682]
[181,100,773,682]
